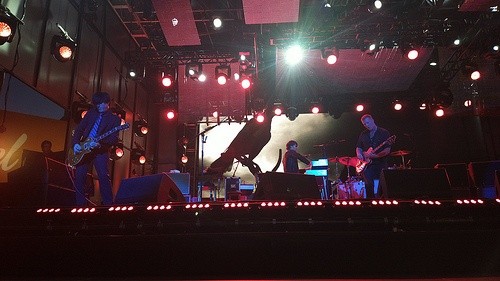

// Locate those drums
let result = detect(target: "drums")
[323,179,338,199]
[345,177,366,199]
[336,184,350,200]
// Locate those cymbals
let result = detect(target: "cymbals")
[338,156,355,168]
[328,157,340,163]
[389,150,413,157]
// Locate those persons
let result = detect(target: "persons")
[41,140,56,162]
[356,114,390,198]
[283,140,311,174]
[71,93,121,206]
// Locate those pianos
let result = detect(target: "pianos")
[304,158,329,200]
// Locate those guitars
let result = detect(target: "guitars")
[355,134,397,174]
[67,123,131,169]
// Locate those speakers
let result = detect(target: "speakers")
[114,172,189,205]
[466,160,500,197]
[250,170,321,200]
[378,167,455,201]
